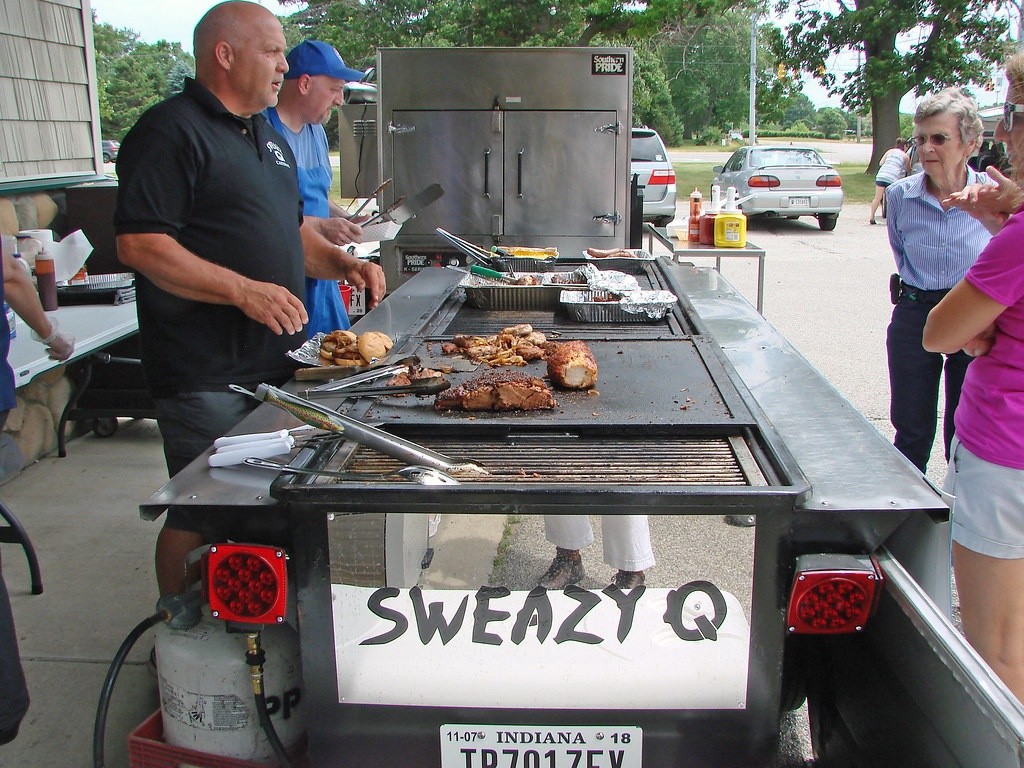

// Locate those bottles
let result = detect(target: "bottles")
[33,246,59,311]
[11,253,32,283]
[712,210,747,247]
[3,300,17,340]
[688,198,702,243]
[689,187,703,219]
[698,210,721,245]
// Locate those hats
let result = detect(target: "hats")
[283,40,366,80]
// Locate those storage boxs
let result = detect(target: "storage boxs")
[126,709,273,768]
[501,252,560,272]
[580,249,656,272]
[560,289,678,323]
[86,272,135,289]
[457,269,592,313]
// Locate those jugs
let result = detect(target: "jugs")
[297,354,451,400]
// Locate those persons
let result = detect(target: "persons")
[883,85,1000,477]
[114,0,387,672]
[923,50,1023,705]
[869,137,912,225]
[0,234,75,436]
[262,40,373,344]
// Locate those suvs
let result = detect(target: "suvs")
[102,140,121,163]
[632,124,677,228]
[881,105,1011,217]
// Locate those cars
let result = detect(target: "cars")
[842,130,857,135]
[710,146,845,231]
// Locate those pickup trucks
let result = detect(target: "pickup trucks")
[729,130,743,140]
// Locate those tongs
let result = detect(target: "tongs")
[348,177,407,228]
[252,382,487,474]
[435,227,510,273]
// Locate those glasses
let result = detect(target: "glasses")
[1004,101,1024,130]
[907,134,978,146]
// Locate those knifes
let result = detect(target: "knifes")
[214,420,382,449]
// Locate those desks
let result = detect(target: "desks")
[0,300,146,595]
[647,222,768,316]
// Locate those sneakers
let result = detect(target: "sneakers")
[536,555,584,590]
[611,567,645,589]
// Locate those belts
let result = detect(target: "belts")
[900,281,944,304]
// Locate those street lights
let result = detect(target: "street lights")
[995,91,1001,105]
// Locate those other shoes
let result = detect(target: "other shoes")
[0,693,30,745]
[428,514,442,537]
[870,220,876,225]
